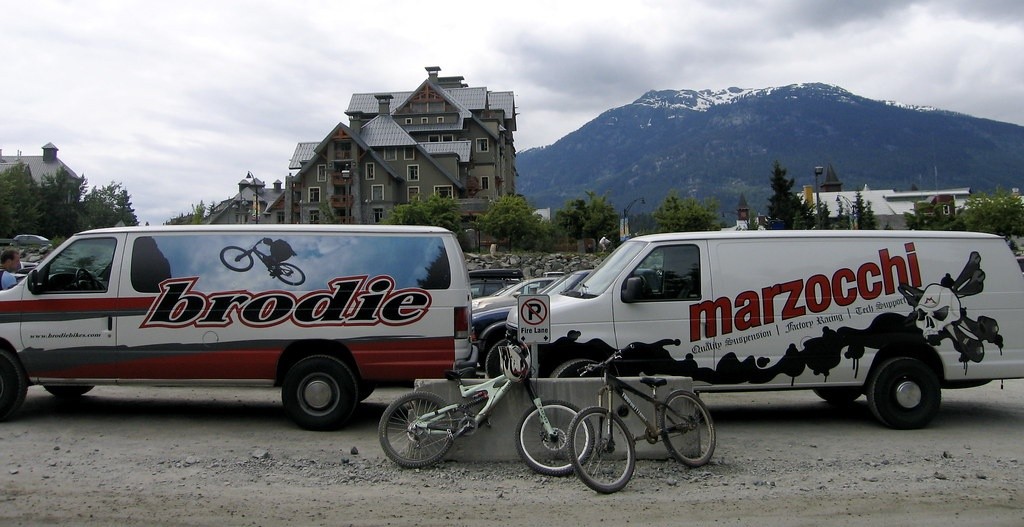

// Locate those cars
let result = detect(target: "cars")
[467,268,665,373]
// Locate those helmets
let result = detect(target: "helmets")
[498,340,531,384]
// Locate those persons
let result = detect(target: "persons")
[0,250,21,291]
[599,234,611,252]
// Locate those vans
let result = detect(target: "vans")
[0,224,479,432]
[504,229,1024,431]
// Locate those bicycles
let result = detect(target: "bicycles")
[566,338,718,494]
[377,326,595,477]
